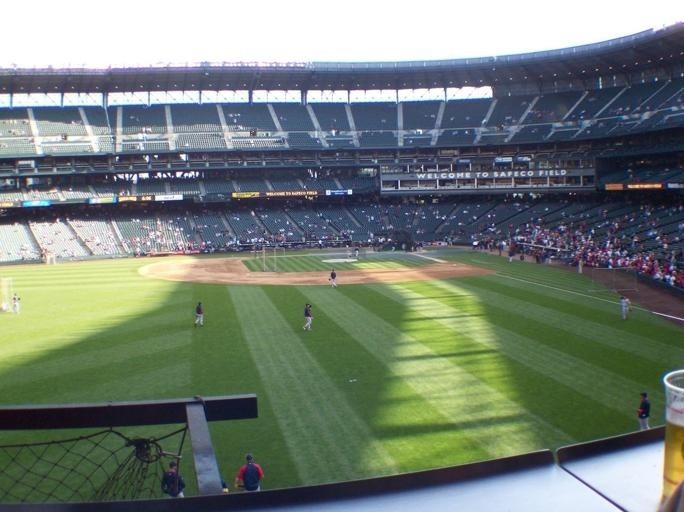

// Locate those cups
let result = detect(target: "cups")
[661,367,684,499]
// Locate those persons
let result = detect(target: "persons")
[9,292,21,314]
[193,300,204,328]
[620,294,634,322]
[1,93,684,291]
[302,303,315,333]
[634,393,652,430]
[160,461,187,498]
[234,454,265,492]
